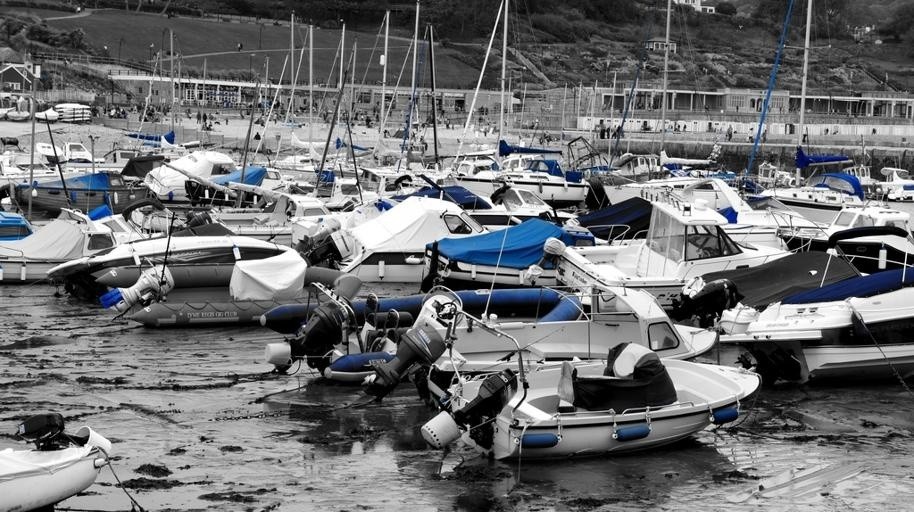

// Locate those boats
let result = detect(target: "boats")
[362,122,450,187]
[306,144,403,183]
[90,263,363,327]
[260,273,585,382]
[376,180,576,228]
[349,187,581,233]
[145,150,255,201]
[872,167,914,203]
[567,175,816,243]
[682,226,914,387]
[419,312,763,463]
[566,136,661,178]
[779,205,914,277]
[540,187,795,320]
[768,146,889,212]
[131,196,333,236]
[268,155,363,187]
[123,182,377,250]
[619,177,809,227]
[280,173,381,211]
[420,218,613,295]
[361,284,718,410]
[0,137,102,189]
[17,170,150,216]
[767,188,914,252]
[421,357,760,464]
[717,227,914,393]
[840,164,881,198]
[260,284,718,397]
[659,150,774,204]
[451,154,589,210]
[46,234,364,328]
[555,185,830,310]
[367,246,719,405]
[13,149,267,216]
[98,133,194,173]
[423,221,630,290]
[565,197,652,238]
[46,221,306,305]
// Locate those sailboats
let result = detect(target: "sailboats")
[769,0,886,208]
